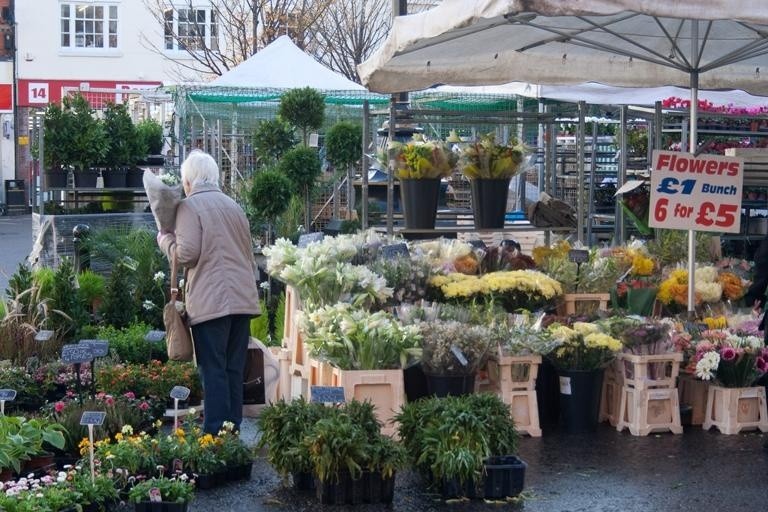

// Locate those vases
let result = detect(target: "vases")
[468,177,512,230]
[701,388,768,438]
[397,177,443,231]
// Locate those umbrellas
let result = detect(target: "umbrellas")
[356,0,768,325]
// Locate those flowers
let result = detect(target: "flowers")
[371,132,461,179]
[656,94,768,156]
[446,127,529,179]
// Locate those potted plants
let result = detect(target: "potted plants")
[249,395,414,507]
[128,114,166,190]
[62,93,112,190]
[30,101,70,189]
[101,100,149,189]
[388,393,529,502]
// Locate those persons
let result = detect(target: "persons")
[156,147,264,436]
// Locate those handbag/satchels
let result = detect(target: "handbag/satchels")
[528,198,577,233]
[163,304,191,362]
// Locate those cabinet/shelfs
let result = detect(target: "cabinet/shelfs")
[515,96,768,226]
